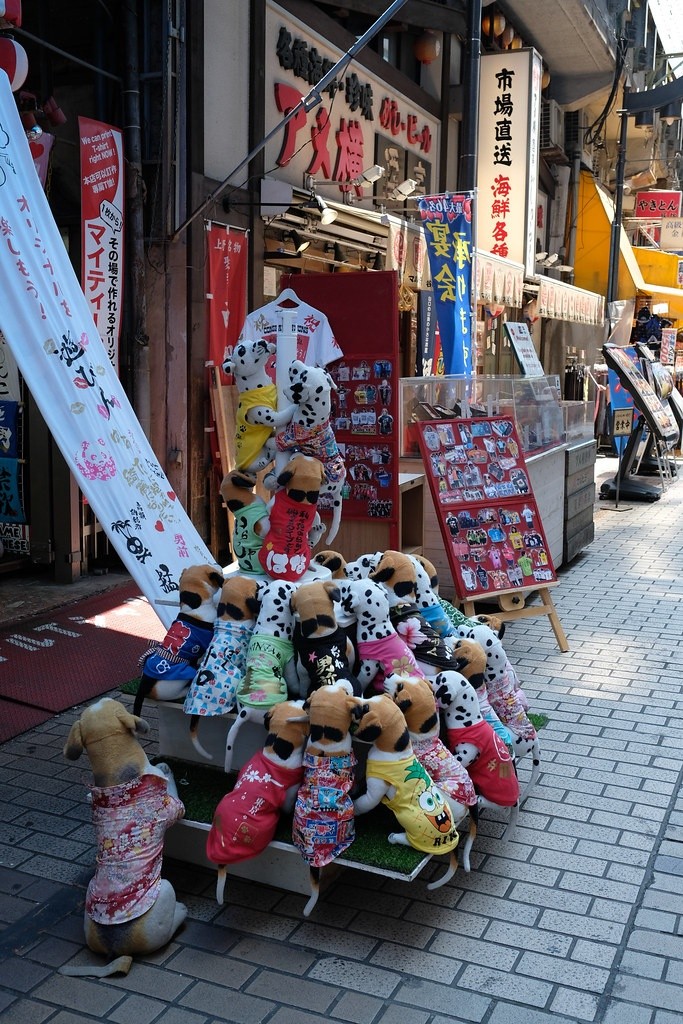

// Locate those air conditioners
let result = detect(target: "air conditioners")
[540,98,565,157]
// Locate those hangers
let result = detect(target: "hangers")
[274,274,303,305]
[637,303,673,326]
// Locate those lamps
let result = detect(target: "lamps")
[306,164,385,192]
[536,253,558,266]
[343,179,416,205]
[16,90,68,143]
[367,251,384,270]
[223,195,338,225]
[623,185,631,195]
[543,265,574,272]
[322,241,349,264]
[535,252,548,261]
[283,230,311,253]
